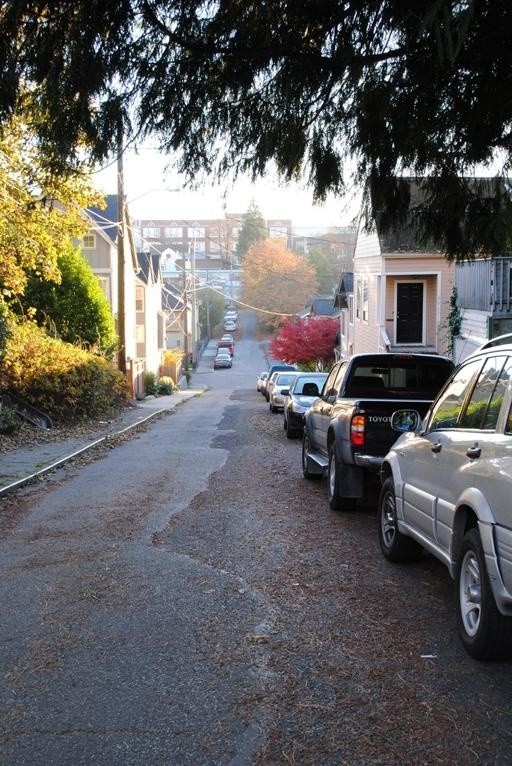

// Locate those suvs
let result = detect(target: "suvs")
[372,330,512,654]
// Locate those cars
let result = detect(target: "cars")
[212,303,240,369]
[255,364,329,440]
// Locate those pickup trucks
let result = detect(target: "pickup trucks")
[297,350,457,511]
